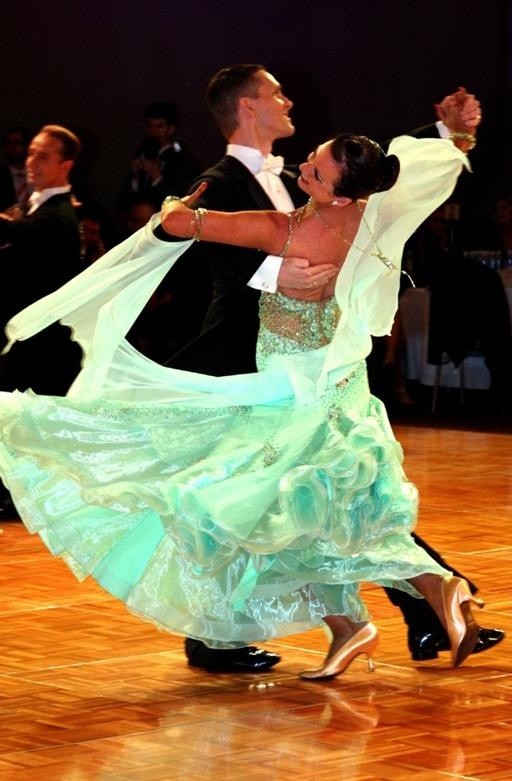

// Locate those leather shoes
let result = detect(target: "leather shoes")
[183,639,284,672]
[406,623,506,660]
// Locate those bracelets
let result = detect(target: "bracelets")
[444,129,480,152]
[158,194,181,211]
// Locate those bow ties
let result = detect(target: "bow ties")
[259,153,286,177]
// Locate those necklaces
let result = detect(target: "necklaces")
[307,195,417,288]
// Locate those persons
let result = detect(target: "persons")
[126,63,506,673]
[0,67,512,529]
[158,84,487,685]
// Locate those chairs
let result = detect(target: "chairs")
[430,256,511,411]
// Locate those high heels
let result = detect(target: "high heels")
[297,621,379,679]
[441,577,485,667]
